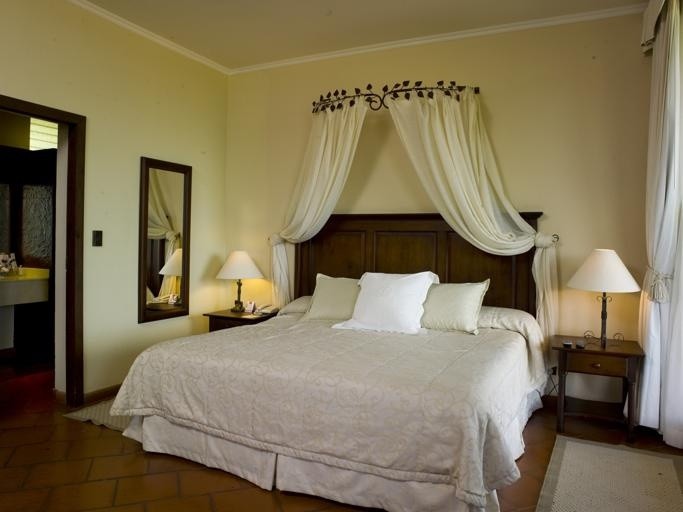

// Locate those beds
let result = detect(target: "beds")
[112,211,550,512]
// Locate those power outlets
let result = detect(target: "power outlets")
[93,230,103,246]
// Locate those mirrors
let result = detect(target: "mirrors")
[137,156,192,322]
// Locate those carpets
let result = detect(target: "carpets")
[64,399,133,433]
[537,433,683,512]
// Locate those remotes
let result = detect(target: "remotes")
[576,339,584,349]
[561,339,573,347]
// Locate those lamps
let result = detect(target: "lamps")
[567,249,642,351]
[215,252,266,314]
[158,248,184,305]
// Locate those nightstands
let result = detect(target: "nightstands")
[547,335,645,443]
[203,308,278,334]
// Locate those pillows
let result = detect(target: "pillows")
[417,277,491,336]
[306,271,361,334]
[332,271,440,335]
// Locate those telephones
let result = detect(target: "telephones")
[254,304,279,316]
[152,294,170,303]
